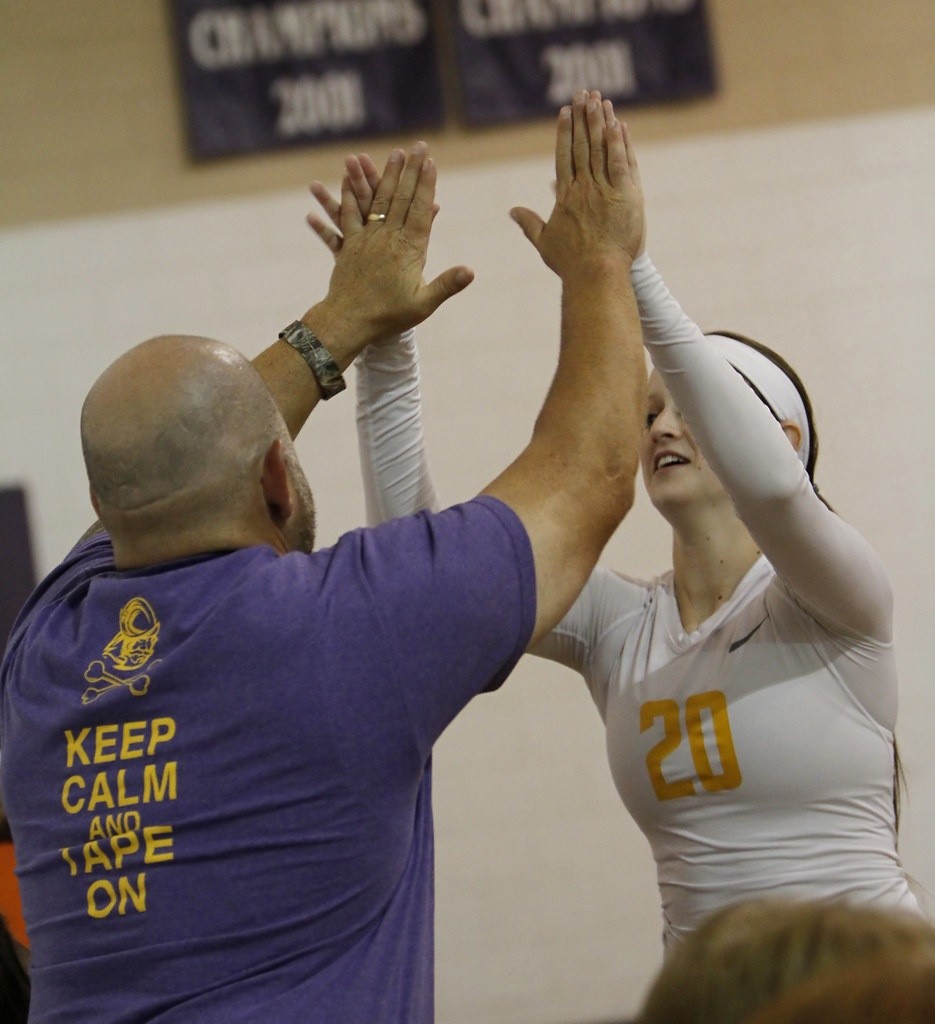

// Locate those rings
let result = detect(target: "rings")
[367,213,386,221]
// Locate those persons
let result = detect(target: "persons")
[0,91,647,1024]
[305,90,925,968]
[636,898,935,1024]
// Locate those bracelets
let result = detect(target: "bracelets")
[278,320,347,401]
